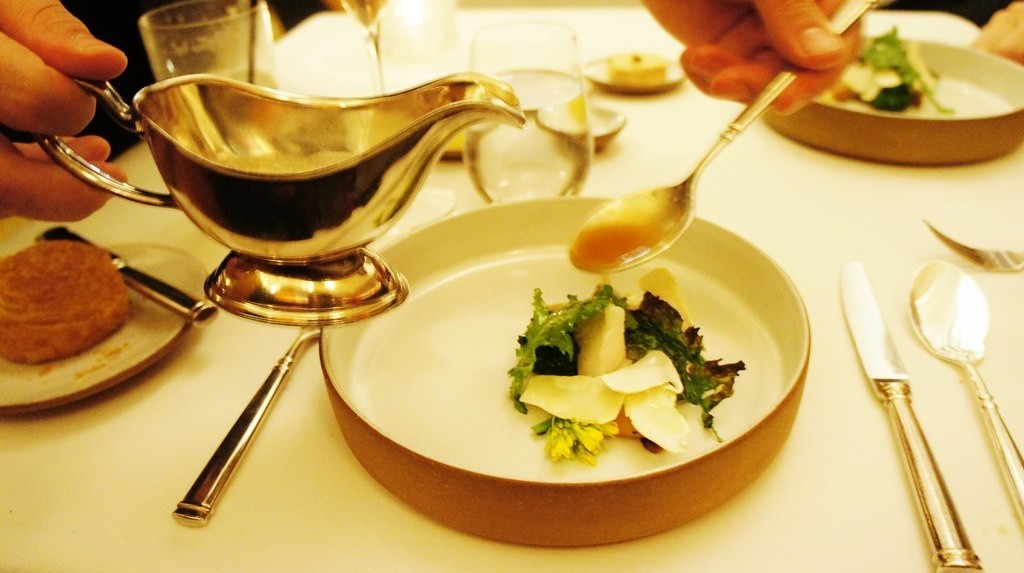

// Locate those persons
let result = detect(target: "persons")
[0,0,862,222]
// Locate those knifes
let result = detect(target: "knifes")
[34,226,219,328]
[840,261,984,573]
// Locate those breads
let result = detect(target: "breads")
[0,240,130,364]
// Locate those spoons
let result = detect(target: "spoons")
[908,262,1024,536]
[569,0,879,277]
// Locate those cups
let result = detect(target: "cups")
[140,0,281,92]
[463,24,594,205]
[36,57,523,325]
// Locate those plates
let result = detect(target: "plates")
[487,66,592,113]
[0,244,209,415]
[538,106,627,150]
[581,50,689,96]
[762,34,1024,168]
[320,198,811,550]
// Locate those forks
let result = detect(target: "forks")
[922,218,1024,274]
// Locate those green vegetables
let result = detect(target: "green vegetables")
[506,282,745,468]
[854,24,953,115]
